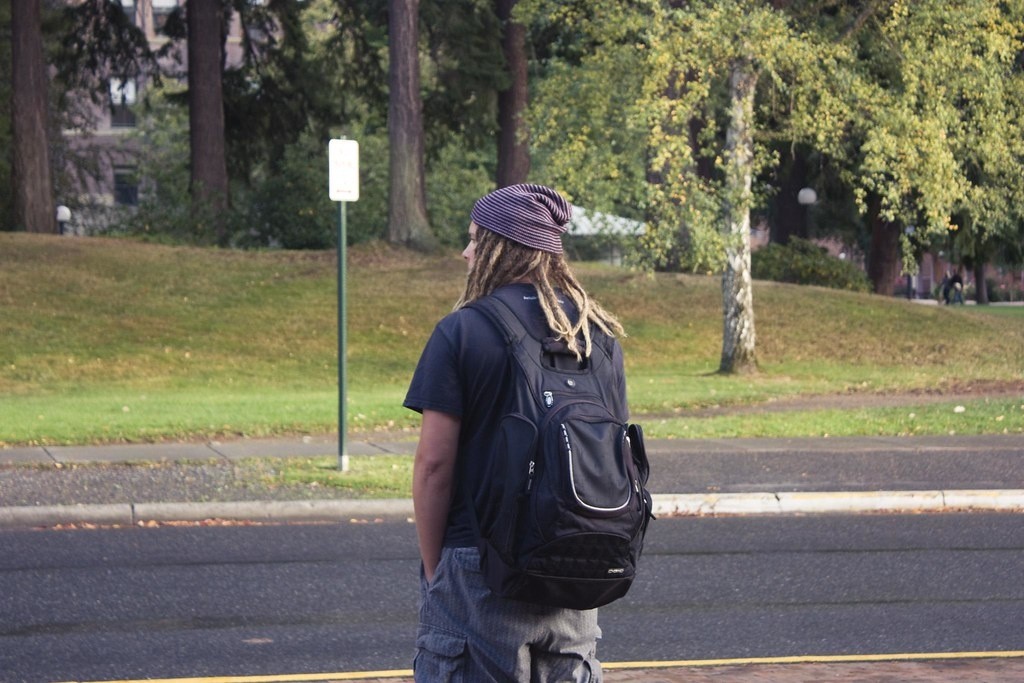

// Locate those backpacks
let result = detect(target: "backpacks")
[461,296,656,610]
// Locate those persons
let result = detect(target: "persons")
[938,269,965,306]
[404,185,630,683]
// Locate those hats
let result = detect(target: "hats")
[470,184,572,254]
